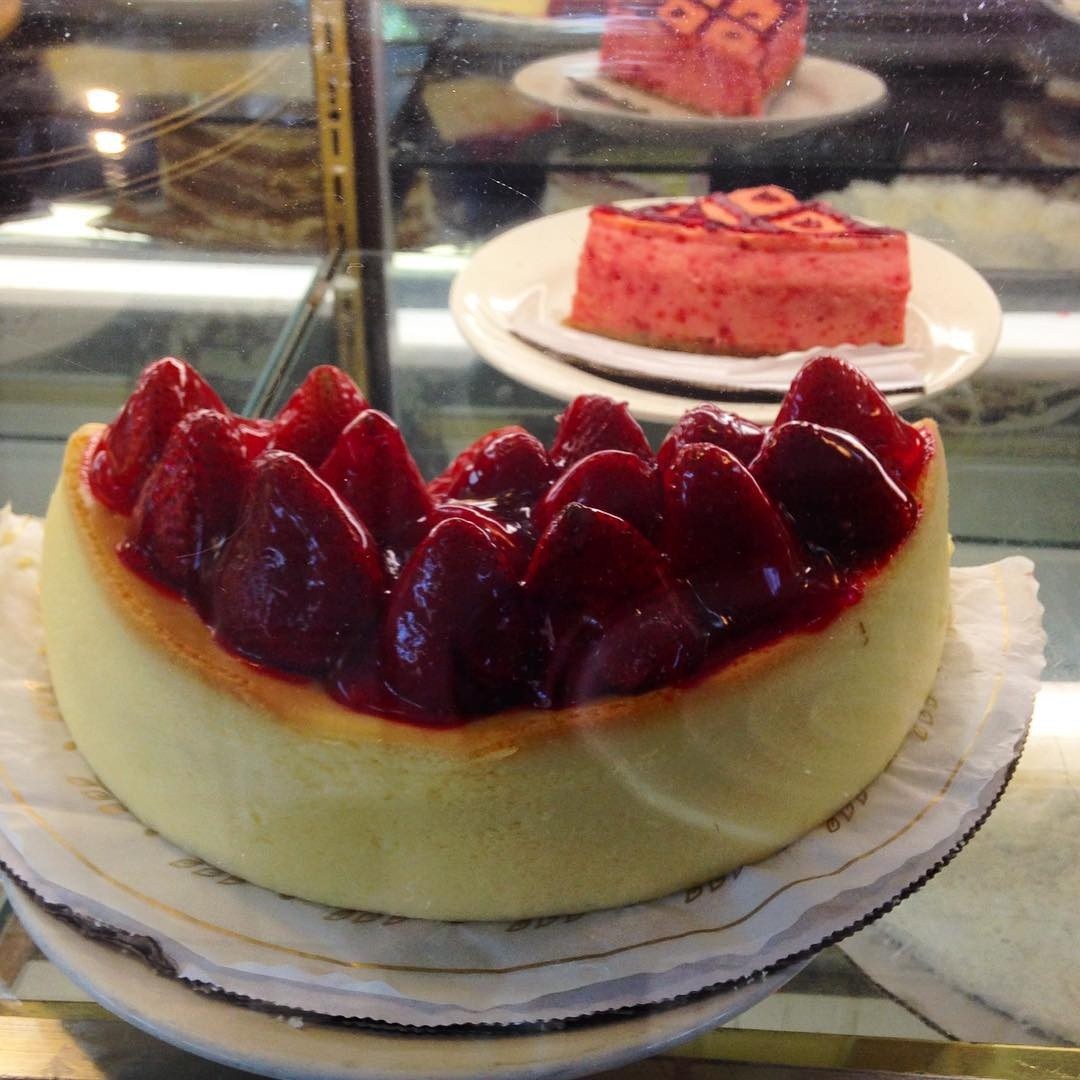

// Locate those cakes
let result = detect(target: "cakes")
[38,357,952,923]
[599,0,810,121]
[156,111,321,246]
[560,182,915,357]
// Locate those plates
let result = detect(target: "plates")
[456,10,609,48]
[0,868,826,1080]
[446,191,1003,428]
[510,47,891,143]
